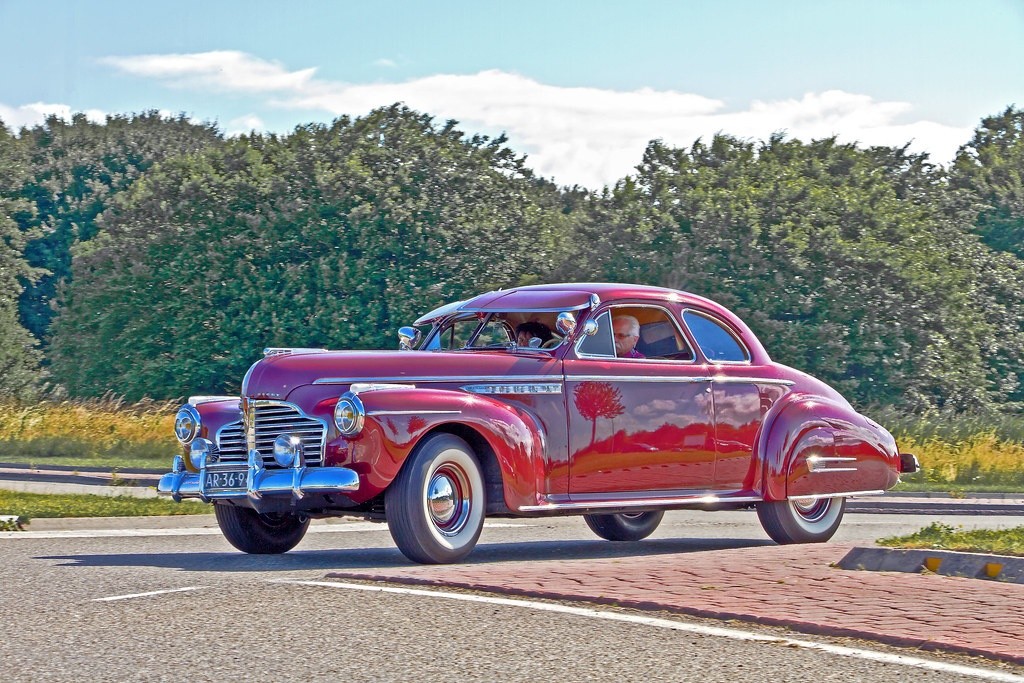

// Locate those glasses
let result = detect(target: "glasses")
[613,333,633,338]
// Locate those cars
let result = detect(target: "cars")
[157,281,920,566]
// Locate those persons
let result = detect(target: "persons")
[515,323,554,349]
[612,315,646,359]
